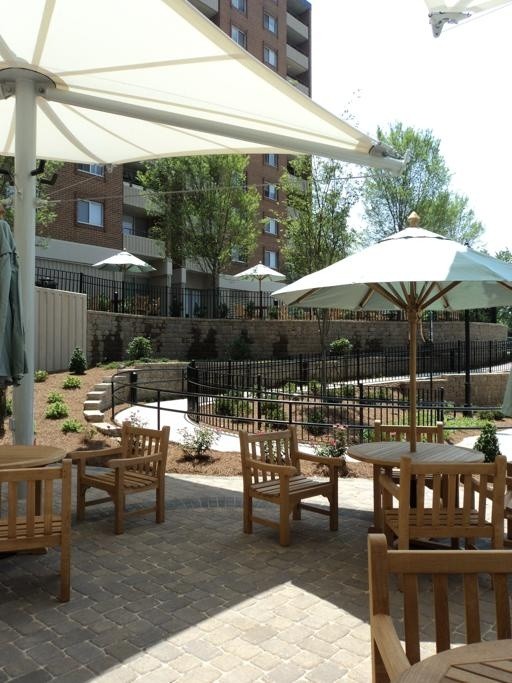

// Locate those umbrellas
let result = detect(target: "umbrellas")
[271,213,512,480]
[0,0,399,500]
[234,259,285,318]
[88,244,154,311]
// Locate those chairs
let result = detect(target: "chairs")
[70,421,170,535]
[0,460,73,602]
[136,297,346,319]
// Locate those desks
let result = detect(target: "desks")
[0,444,67,552]
